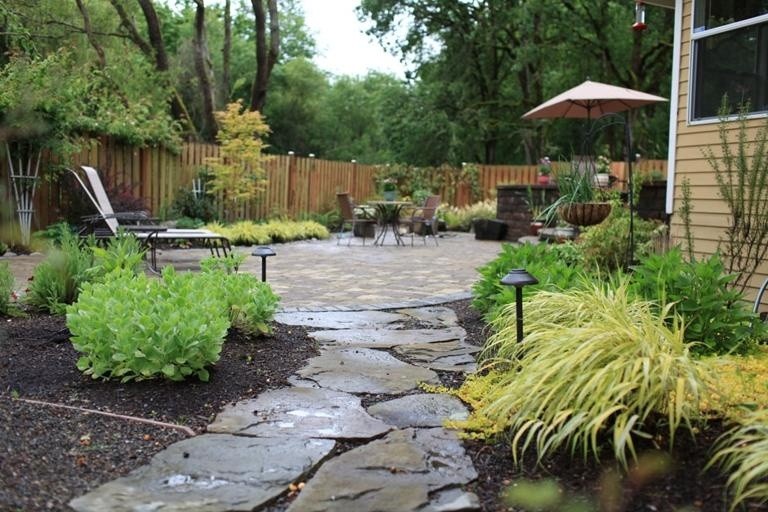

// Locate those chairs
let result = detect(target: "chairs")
[58,163,238,278]
[397,196,441,246]
[80,166,221,260]
[336,192,376,247]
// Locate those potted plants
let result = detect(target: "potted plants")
[534,139,620,233]
[627,168,667,221]
[521,184,546,236]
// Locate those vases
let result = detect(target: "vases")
[538,174,550,185]
[384,190,396,201]
[594,173,609,187]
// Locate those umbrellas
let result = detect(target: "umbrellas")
[521,75,670,155]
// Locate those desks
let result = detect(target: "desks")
[367,200,413,246]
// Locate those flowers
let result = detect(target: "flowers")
[536,157,553,176]
[596,154,611,173]
[384,177,397,192]
